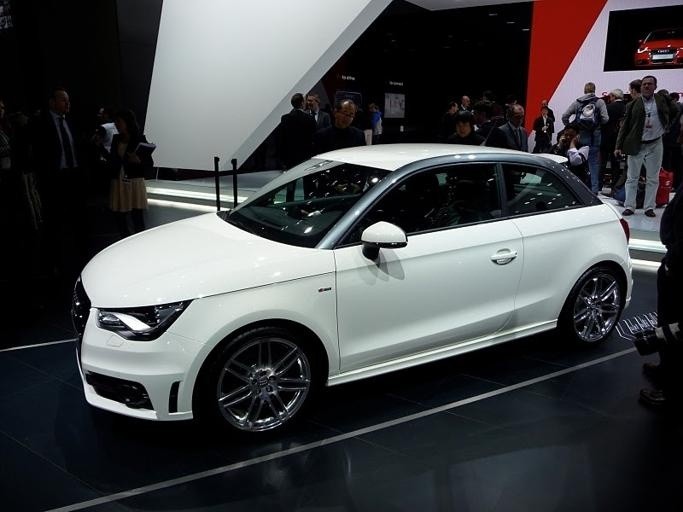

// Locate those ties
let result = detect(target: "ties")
[311,112,316,121]
[515,128,522,148]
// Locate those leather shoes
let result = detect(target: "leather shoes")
[600,192,612,197]
[634,326,661,356]
[622,209,634,215]
[645,209,656,217]
[640,362,668,409]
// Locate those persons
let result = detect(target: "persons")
[1,86,156,235]
[274,89,385,173]
[639,177,683,415]
[442,77,683,215]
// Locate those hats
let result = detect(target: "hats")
[611,89,624,99]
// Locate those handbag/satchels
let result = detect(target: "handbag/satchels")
[612,171,645,204]
[569,160,591,188]
[657,168,673,205]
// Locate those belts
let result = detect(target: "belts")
[641,137,660,144]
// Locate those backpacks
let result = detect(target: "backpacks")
[578,98,599,127]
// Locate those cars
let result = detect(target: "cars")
[637,28,683,68]
[72,142,632,438]
